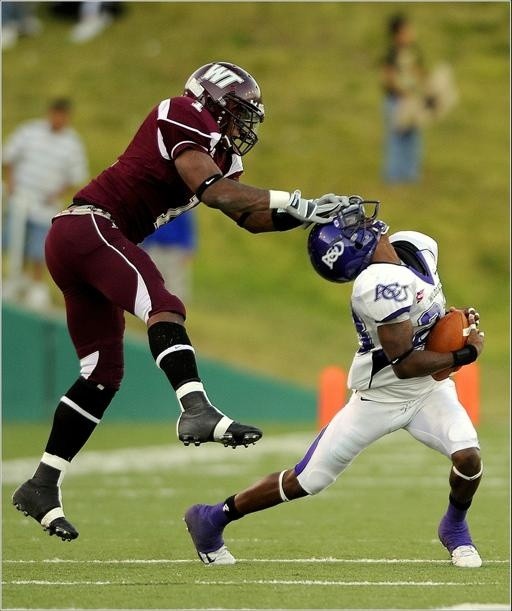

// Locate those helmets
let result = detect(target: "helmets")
[184,62,265,156]
[308,195,389,283]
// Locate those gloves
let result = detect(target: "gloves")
[269,189,350,232]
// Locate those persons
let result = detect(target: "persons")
[50,2,126,43]
[4,92,91,309]
[379,9,429,184]
[10,60,351,543]
[180,196,485,571]
[2,3,46,47]
[141,209,199,310]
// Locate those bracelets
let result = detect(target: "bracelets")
[269,190,291,209]
[271,208,304,232]
[449,345,477,368]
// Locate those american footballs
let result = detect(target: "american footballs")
[425,311,469,380]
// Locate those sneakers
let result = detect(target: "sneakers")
[439,520,482,568]
[11,479,78,542]
[177,407,262,449]
[182,504,236,566]
